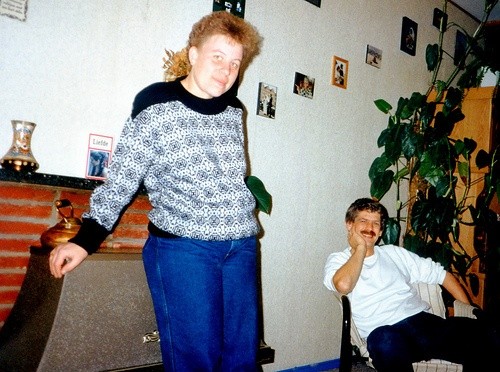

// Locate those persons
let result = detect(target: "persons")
[260,28,416,116]
[90,151,110,180]
[50,12,265,372]
[323,198,500,372]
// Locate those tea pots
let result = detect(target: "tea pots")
[40,199,82,248]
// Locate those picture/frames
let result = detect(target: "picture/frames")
[399,16,419,56]
[331,56,349,90]
[433,8,448,32]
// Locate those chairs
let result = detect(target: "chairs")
[338,281,487,372]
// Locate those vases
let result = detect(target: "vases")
[0,119,41,172]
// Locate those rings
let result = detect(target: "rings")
[50,253,55,256]
